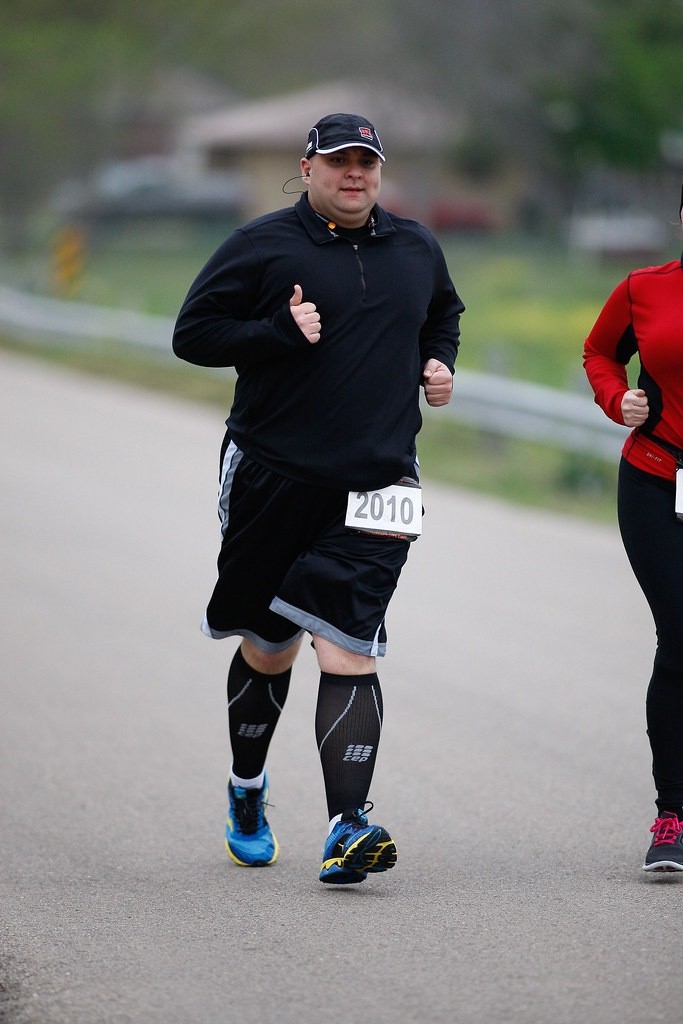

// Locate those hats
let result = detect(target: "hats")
[305,112,386,162]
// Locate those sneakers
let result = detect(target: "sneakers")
[226,768,277,869]
[642,811,683,872]
[319,809,397,884]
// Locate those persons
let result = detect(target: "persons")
[173,114,469,885]
[583,198,683,876]
